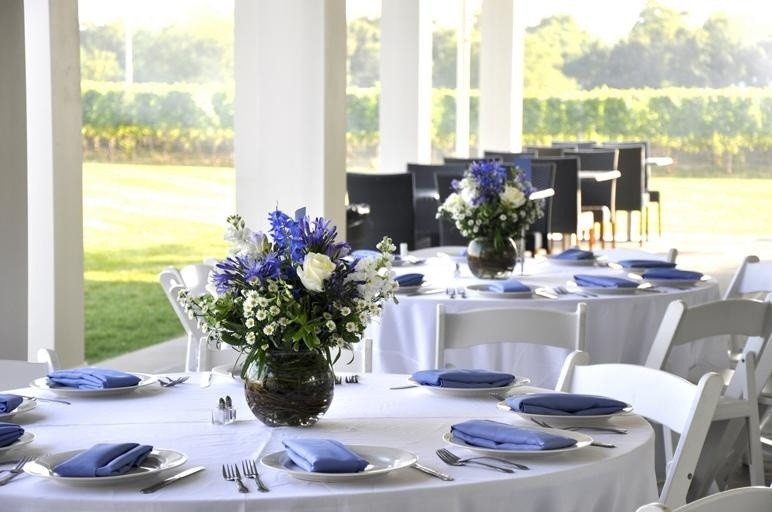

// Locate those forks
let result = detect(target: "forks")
[159,374,191,388]
[1,454,38,476]
[528,413,629,447]
[335,373,360,386]
[444,284,468,302]
[436,448,527,473]
[552,286,597,297]
[241,458,268,492]
[222,464,250,493]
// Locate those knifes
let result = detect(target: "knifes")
[391,384,418,390]
[22,393,71,406]
[140,465,206,495]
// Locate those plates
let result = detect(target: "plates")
[356,253,716,300]
[0,432,36,451]
[29,370,160,397]
[442,427,593,457]
[24,446,188,486]
[262,445,418,481]
[409,373,533,398]
[497,396,635,424]
[211,364,245,381]
[1,398,37,420]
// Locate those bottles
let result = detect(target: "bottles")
[213,394,237,424]
